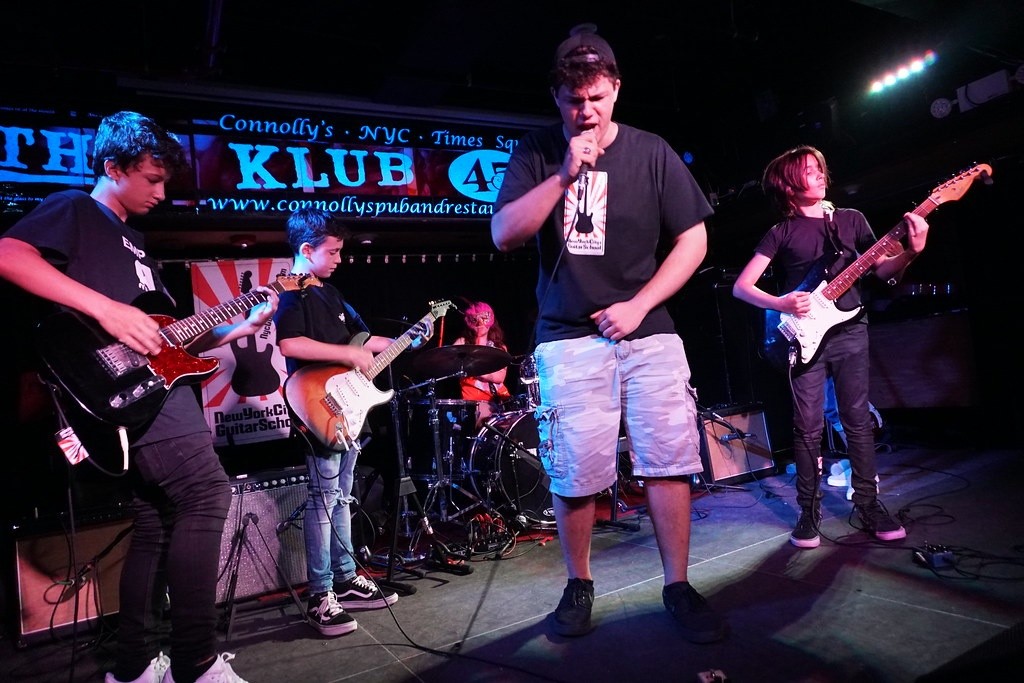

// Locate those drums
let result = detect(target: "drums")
[468,409,557,524]
[403,397,478,482]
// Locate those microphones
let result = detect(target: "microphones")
[577,129,597,200]
[721,433,756,442]
[276,521,293,533]
[59,576,87,588]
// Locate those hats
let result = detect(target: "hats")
[554,31,616,72]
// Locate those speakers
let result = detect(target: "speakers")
[210,464,310,605]
[695,403,778,487]
[5,505,142,648]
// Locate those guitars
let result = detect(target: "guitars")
[752,159,994,371]
[30,270,324,437]
[281,297,458,453]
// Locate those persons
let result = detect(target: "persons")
[733,146,931,548]
[453,302,512,430]
[273,209,435,636]
[0,110,281,683]
[491,30,728,643]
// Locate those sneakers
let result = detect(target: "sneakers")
[662,581,724,643]
[854,499,906,540]
[333,575,398,609]
[105,650,175,683]
[306,590,357,635]
[194,652,249,683]
[790,504,823,547]
[554,577,594,635]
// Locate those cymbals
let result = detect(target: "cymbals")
[373,315,414,327]
[413,344,513,378]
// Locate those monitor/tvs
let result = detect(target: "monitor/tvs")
[354,360,559,579]
